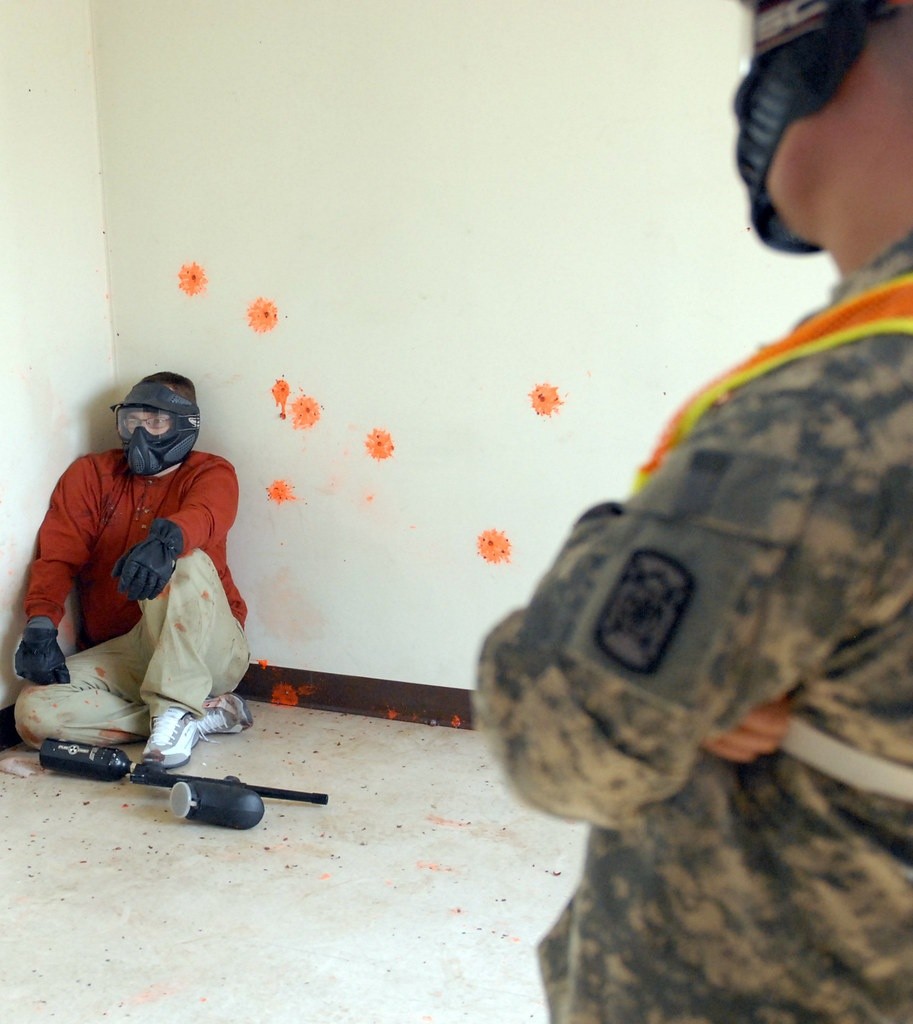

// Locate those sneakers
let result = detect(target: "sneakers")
[197,693,253,741]
[142,708,200,769]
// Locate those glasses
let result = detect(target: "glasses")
[121,416,171,429]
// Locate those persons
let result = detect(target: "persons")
[13,372,253,769]
[472,0,913,1024]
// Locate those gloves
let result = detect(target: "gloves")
[116,518,184,601]
[15,625,70,685]
